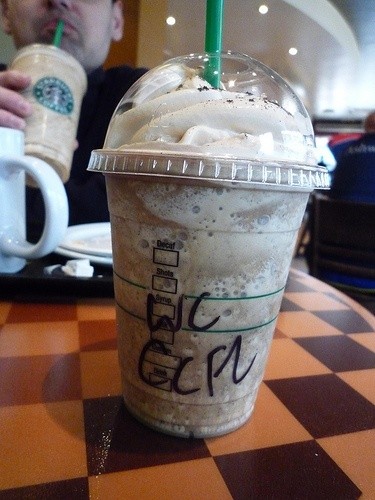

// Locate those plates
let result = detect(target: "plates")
[50,222,114,265]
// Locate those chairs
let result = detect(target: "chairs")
[294,195,375,301]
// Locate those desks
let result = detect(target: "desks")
[0,268,375,500]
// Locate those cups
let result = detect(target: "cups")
[6,43,85,188]
[86,52,330,441]
[0,126,69,273]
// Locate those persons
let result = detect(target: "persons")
[303,109,375,308]
[0,0,153,240]
